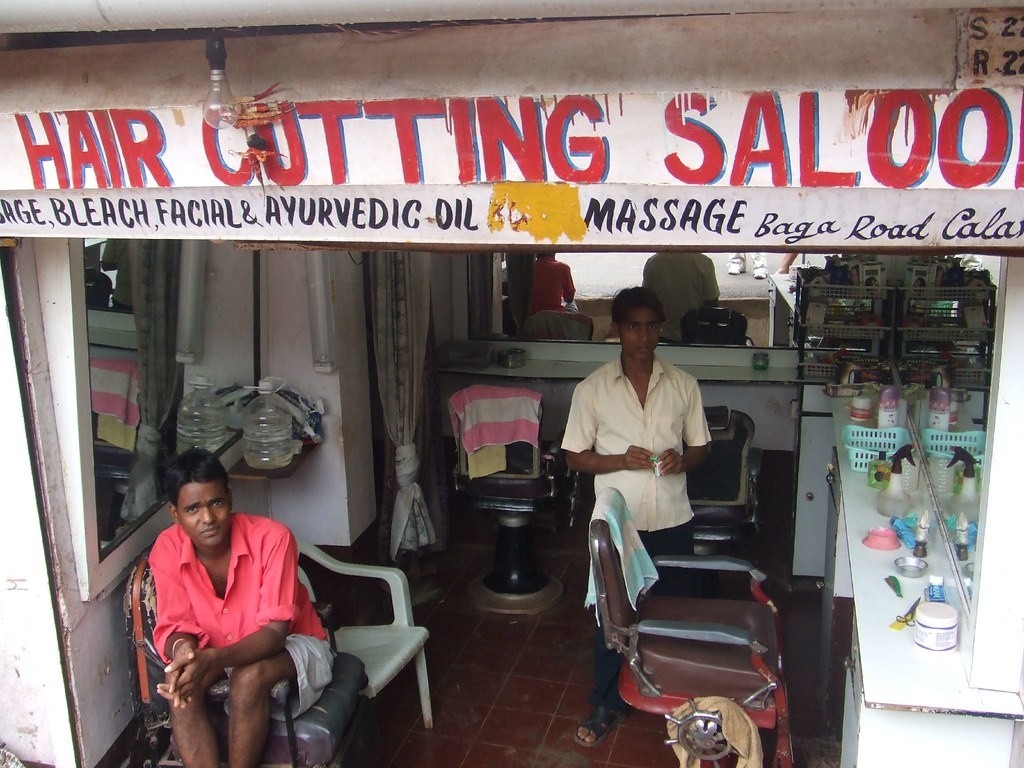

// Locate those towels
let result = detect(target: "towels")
[447,384,542,480]
[523,310,593,340]
[582,487,659,628]
[88,345,141,452]
[666,695,764,768]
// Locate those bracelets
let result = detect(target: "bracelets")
[172,638,184,660]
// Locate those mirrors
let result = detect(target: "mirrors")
[83,238,134,314]
[466,254,876,352]
[82,238,262,564]
[894,253,1002,616]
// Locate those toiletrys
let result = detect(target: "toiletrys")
[950,402,958,425]
[968,521,978,552]
[889,516,917,549]
[953,463,980,495]
[867,451,891,490]
[850,396,871,421]
[928,389,950,432]
[878,385,899,429]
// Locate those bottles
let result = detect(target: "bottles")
[242,375,293,470]
[901,443,920,490]
[929,453,954,499]
[175,375,227,456]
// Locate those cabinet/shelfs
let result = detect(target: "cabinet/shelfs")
[767,274,796,346]
[789,384,1015,768]
[895,278,996,387]
[794,266,893,384]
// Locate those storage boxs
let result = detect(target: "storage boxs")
[905,257,952,300]
[840,259,887,299]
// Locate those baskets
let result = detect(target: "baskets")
[842,424,911,473]
[899,286,992,300]
[804,357,880,376]
[809,283,890,301]
[897,328,995,343]
[802,322,884,338]
[903,361,984,383]
[920,428,986,467]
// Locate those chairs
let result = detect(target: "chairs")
[447,382,589,615]
[522,310,594,341]
[123,535,433,768]
[88,273,112,308]
[88,356,177,541]
[679,305,754,346]
[585,487,793,768]
[681,406,760,597]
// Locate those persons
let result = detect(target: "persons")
[148,449,327,768]
[561,286,712,748]
[101,238,133,309]
[642,252,721,342]
[777,253,799,274]
[529,252,579,315]
[728,252,768,279]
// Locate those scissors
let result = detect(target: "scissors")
[896,597,920,627]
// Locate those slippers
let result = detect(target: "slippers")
[574,706,625,747]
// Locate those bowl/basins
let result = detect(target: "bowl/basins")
[499,348,525,368]
[894,553,928,578]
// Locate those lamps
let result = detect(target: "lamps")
[175,240,209,362]
[305,250,337,373]
